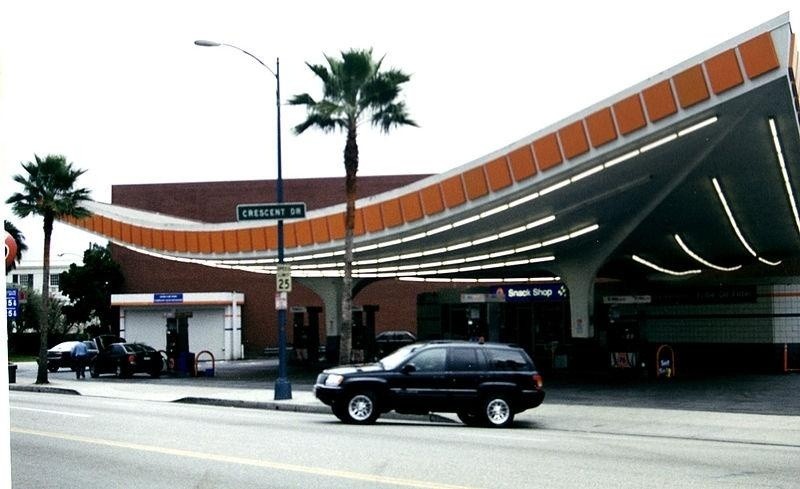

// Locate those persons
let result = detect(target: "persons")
[70,336,88,378]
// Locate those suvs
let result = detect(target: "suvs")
[313,340,543,425]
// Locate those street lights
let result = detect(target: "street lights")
[192,37,295,401]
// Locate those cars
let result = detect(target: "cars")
[44,332,165,380]
